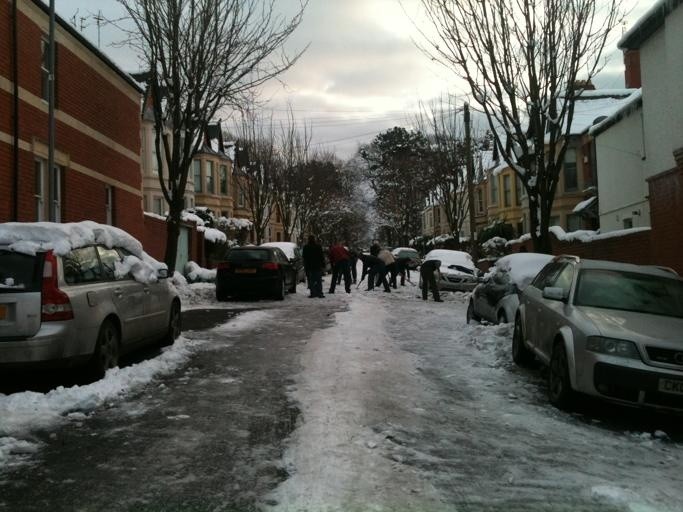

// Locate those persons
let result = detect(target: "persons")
[301,232,447,303]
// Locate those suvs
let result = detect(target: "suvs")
[511,254,683,436]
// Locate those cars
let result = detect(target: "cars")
[0,242,181,379]
[418,249,480,292]
[216,241,332,301]
[466,253,554,325]
[391,247,419,270]
